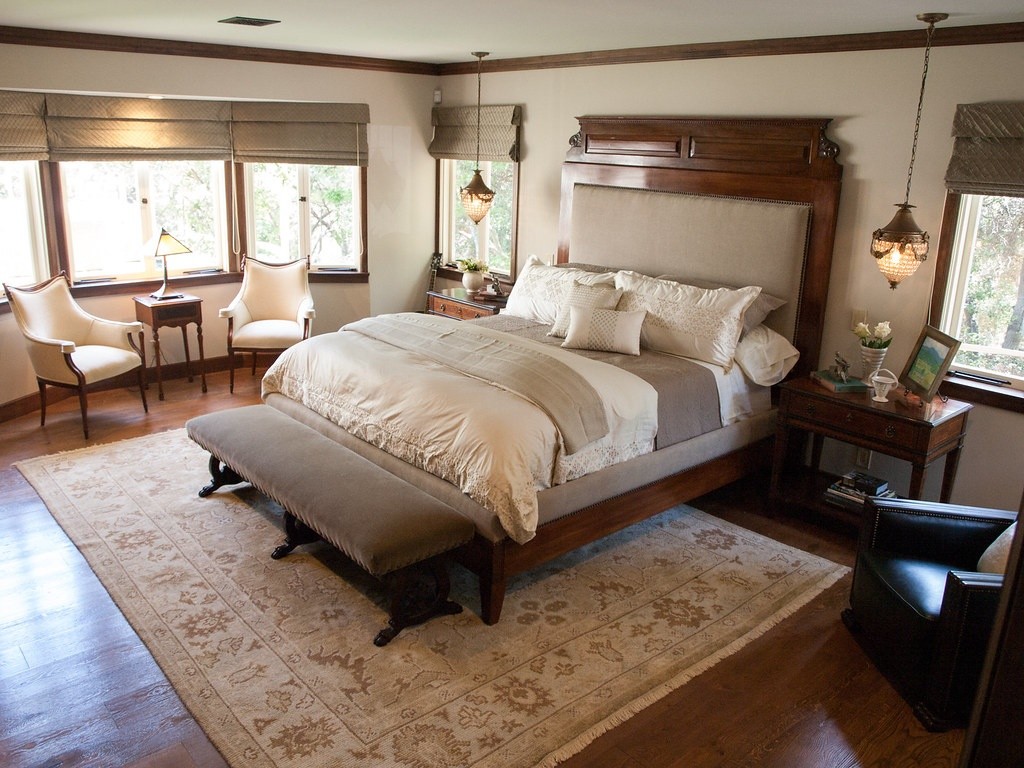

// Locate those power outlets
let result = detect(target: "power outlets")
[855,447,873,470]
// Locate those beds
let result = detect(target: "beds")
[261,115,845,627]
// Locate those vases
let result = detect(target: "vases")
[461,270,484,293]
[859,343,888,388]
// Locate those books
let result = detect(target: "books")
[824,471,898,513]
[473,291,509,302]
[810,371,868,393]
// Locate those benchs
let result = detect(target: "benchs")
[184,403,475,647]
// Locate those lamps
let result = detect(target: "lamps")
[148,225,193,301]
[459,52,498,226]
[869,11,950,291]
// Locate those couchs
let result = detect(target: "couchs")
[840,495,1020,733]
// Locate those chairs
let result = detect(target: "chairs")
[218,254,317,394]
[1,269,149,440]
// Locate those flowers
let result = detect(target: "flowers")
[455,257,489,274]
[853,320,893,349]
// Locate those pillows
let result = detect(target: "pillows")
[976,521,1017,574]
[503,257,617,325]
[613,269,762,375]
[554,263,661,279]
[545,278,623,339]
[560,305,648,357]
[665,276,788,343]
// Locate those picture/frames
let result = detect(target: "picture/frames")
[898,324,962,404]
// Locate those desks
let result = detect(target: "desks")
[131,291,208,401]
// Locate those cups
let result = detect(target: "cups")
[871,376,895,403]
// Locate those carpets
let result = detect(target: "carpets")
[10,426,854,768]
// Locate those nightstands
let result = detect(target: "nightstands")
[766,374,976,528]
[425,287,506,321]
